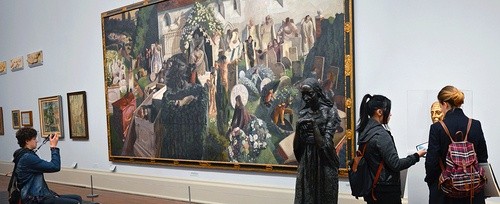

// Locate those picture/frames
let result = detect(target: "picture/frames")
[12,110,20,129]
[101,0,355,177]
[21,111,33,126]
[38,95,64,138]
[67,91,88,139]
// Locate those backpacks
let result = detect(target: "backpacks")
[440,118,486,198]
[348,143,385,200]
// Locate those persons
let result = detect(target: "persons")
[292,78,341,204]
[431,101,444,124]
[424,86,491,204]
[12,127,82,204]
[355,93,428,204]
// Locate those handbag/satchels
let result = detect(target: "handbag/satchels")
[8,172,23,204]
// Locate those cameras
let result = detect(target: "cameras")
[48,134,55,140]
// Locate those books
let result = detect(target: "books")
[479,163,500,197]
